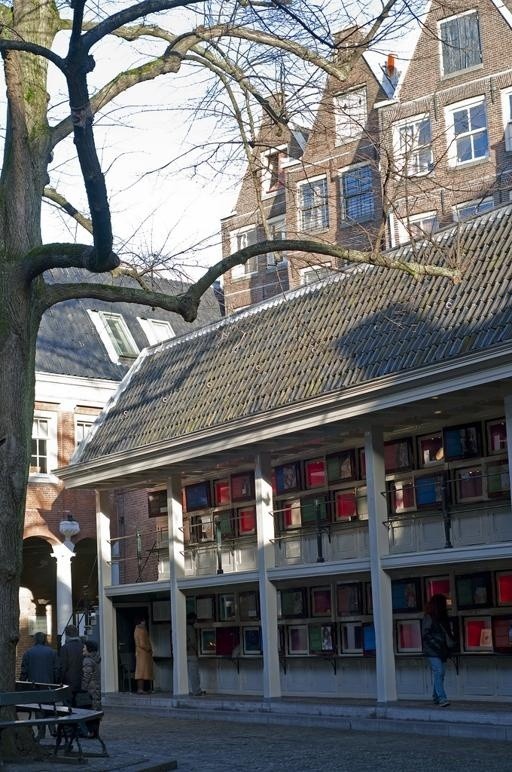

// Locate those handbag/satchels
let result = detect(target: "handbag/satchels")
[76,691,92,707]
[445,633,457,649]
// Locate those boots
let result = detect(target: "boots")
[137,679,149,694]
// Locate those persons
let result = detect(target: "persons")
[405,583,416,609]
[186,611,207,696]
[422,594,454,706]
[133,617,155,694]
[322,627,333,650]
[20,632,62,739]
[60,625,91,737]
[81,639,102,738]
[283,467,296,489]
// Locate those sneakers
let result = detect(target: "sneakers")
[431,696,450,707]
[195,690,208,696]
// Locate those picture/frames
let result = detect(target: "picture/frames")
[391,575,422,613]
[425,571,453,609]
[492,612,511,654]
[213,478,232,506]
[364,580,374,616]
[190,514,213,544]
[442,421,481,463]
[482,415,507,456]
[356,484,369,522]
[277,495,302,531]
[272,466,278,497]
[362,621,375,656]
[448,462,487,506]
[184,480,211,512]
[335,582,363,618]
[146,489,168,518]
[300,489,331,527]
[325,447,359,485]
[355,446,368,481]
[305,458,329,488]
[484,456,511,502]
[213,508,236,541]
[230,469,254,503]
[341,621,364,655]
[184,517,189,544]
[446,615,462,653]
[415,430,445,470]
[462,613,493,653]
[275,459,301,495]
[492,566,509,607]
[396,618,424,656]
[389,476,417,515]
[381,435,416,477]
[147,582,336,659]
[455,568,490,610]
[413,469,448,511]
[333,486,358,524]
[236,505,256,536]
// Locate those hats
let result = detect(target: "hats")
[429,593,446,609]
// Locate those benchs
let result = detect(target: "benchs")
[1,704,111,767]
